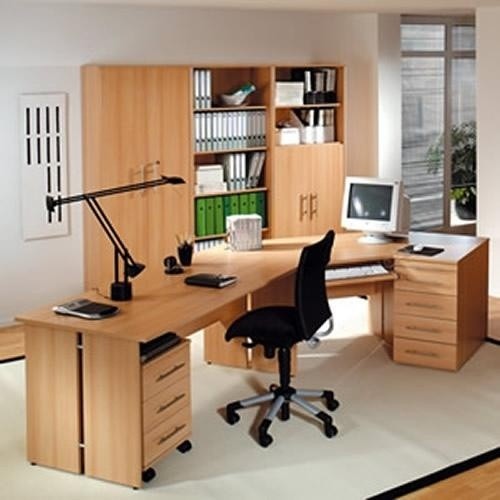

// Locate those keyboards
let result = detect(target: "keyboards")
[325,264,389,281]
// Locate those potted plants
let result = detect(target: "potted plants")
[426,123,482,219]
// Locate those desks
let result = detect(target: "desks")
[12,227,490,489]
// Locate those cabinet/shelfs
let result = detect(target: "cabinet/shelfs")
[83,66,192,291]
[271,64,347,239]
[142,339,192,468]
[393,261,458,370]
[192,66,269,248]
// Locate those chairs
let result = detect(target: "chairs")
[224,228,339,448]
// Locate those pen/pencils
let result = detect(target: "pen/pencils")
[174,231,194,246]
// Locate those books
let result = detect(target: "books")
[275,68,337,106]
[194,69,212,109]
[276,108,335,146]
[225,213,262,251]
[184,272,237,290]
[195,152,266,194]
[194,239,224,254]
[194,111,265,152]
[196,192,266,237]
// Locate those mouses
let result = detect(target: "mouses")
[412,242,424,252]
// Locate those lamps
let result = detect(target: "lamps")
[46,172,185,302]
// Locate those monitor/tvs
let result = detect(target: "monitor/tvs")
[340,176,412,244]
